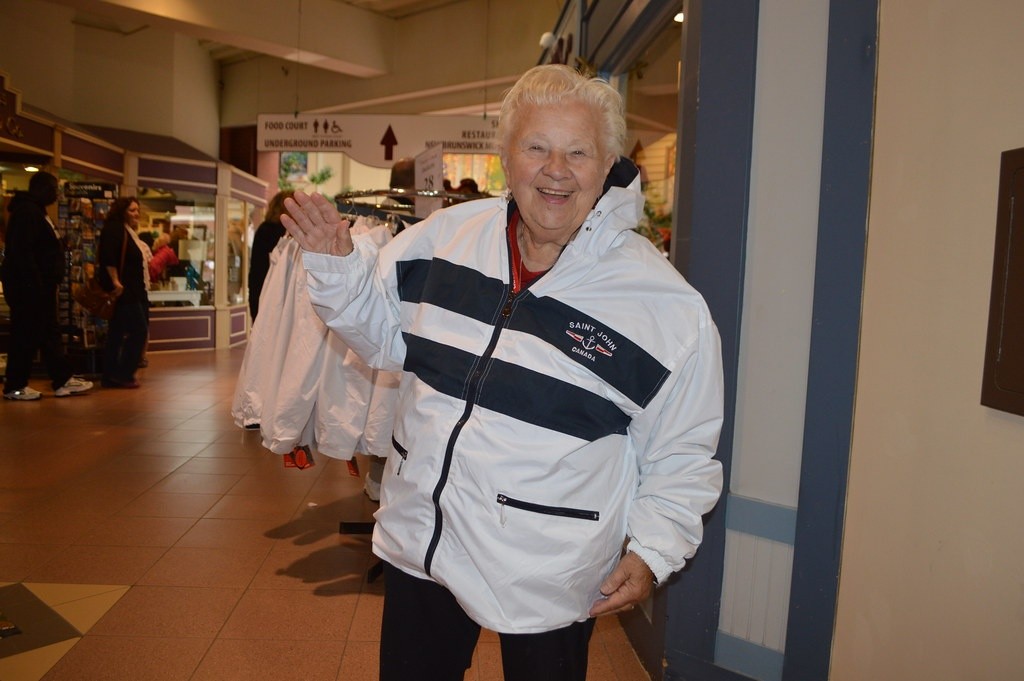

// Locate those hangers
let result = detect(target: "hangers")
[339,190,404,230]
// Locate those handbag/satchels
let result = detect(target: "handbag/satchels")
[75,281,114,320]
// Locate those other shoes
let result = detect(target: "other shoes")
[138,357,148,367]
[101,377,140,388]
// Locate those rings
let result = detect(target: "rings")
[631,604,635,610]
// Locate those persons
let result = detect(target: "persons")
[280,67,724,681]
[244,190,302,431]
[138,224,198,284]
[100,195,148,389]
[443,178,480,203]
[0,170,93,401]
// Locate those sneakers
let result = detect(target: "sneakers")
[55,376,94,396]
[3,386,42,399]
[363,473,382,501]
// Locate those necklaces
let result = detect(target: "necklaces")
[519,223,524,291]
